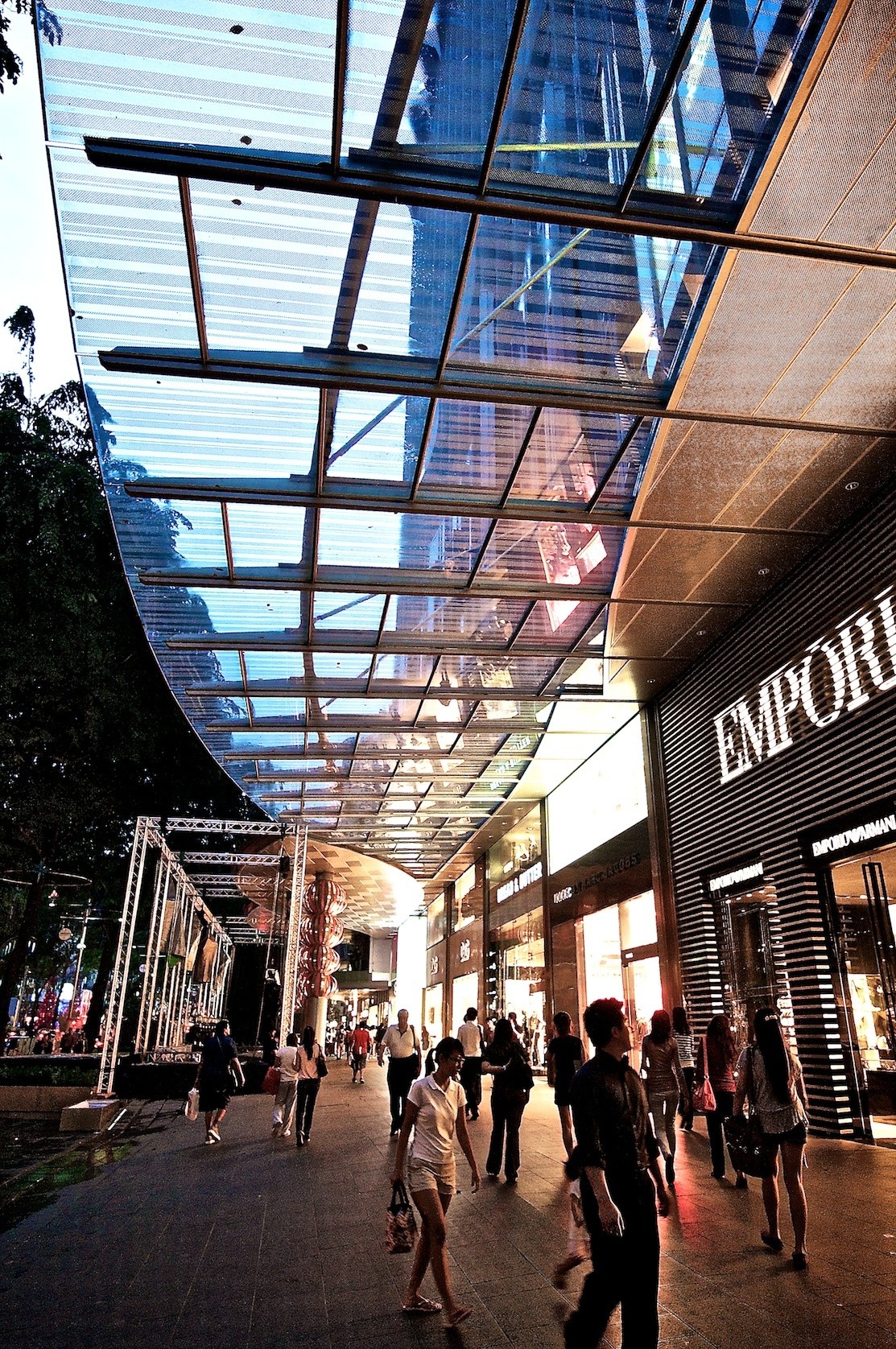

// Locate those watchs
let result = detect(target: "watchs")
[502,1065,506,1071]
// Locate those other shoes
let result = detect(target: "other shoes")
[552,1265,567,1291]
[270,1125,313,1146]
[506,1175,516,1183]
[487,1169,498,1178]
[204,1136,215,1144]
[791,1250,809,1265]
[352,1077,365,1083]
[208,1126,221,1141]
[470,1111,480,1121]
[666,1155,675,1185]
[711,1169,725,1179]
[736,1177,747,1189]
[681,1120,693,1133]
[761,1229,784,1251]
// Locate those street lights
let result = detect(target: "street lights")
[58,898,122,1035]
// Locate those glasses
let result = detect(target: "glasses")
[441,1053,465,1065]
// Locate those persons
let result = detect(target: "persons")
[192,1020,246,1145]
[522,1009,546,1067]
[365,1023,433,1061]
[262,1028,300,1139]
[697,1016,747,1188]
[641,1007,694,1184]
[377,1009,422,1136]
[292,1026,324,1147]
[514,835,538,873]
[553,998,672,1349]
[33,1028,80,1054]
[735,1009,808,1263]
[544,1011,586,1158]
[480,1012,530,1183]
[326,1027,336,1056]
[458,1007,484,1121]
[350,1022,374,1083]
[388,1038,481,1328]
[335,1024,359,1065]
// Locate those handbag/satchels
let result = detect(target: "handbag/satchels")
[690,1076,716,1113]
[385,1178,420,1255]
[184,1088,200,1121]
[317,1043,328,1078]
[723,1114,769,1178]
[409,1024,420,1079]
[260,1063,281,1095]
[225,1064,238,1089]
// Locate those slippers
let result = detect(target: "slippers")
[402,1299,442,1312]
[440,1307,473,1327]
